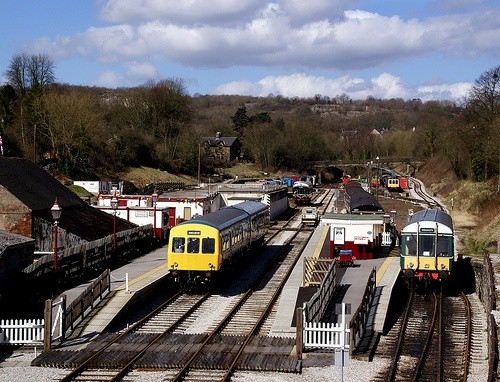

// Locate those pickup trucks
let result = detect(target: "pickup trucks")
[301,207,318,226]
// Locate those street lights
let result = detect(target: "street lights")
[152,189,158,240]
[51,196,62,272]
[112,191,119,254]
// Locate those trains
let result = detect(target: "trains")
[291,182,313,205]
[399,208,454,292]
[167,199,270,295]
